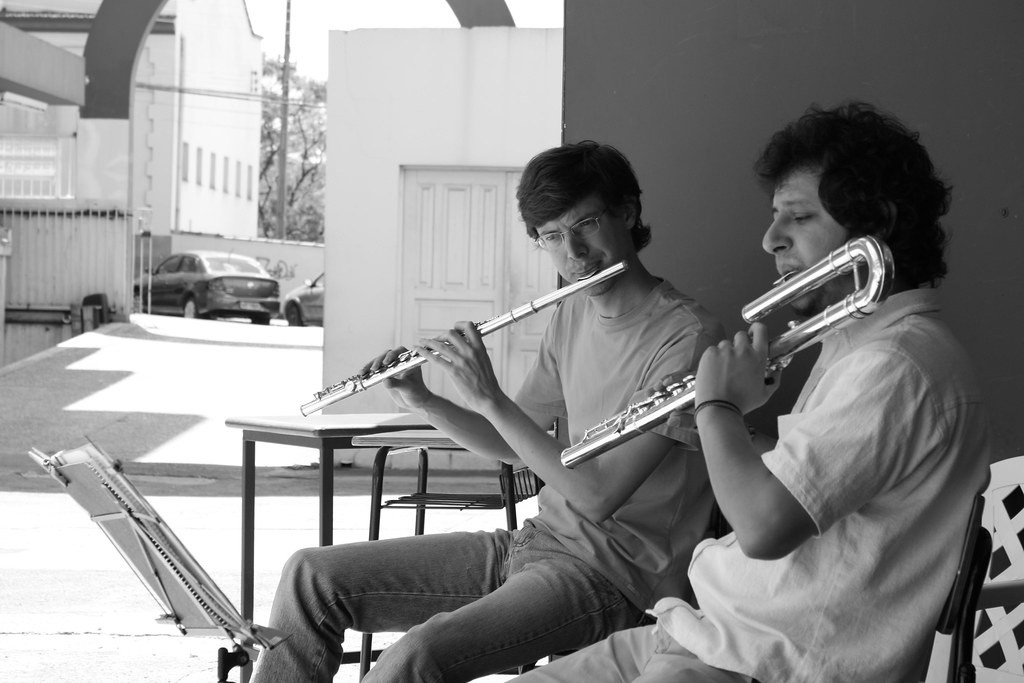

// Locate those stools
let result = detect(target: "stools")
[350,426,561,683]
[225,411,436,640]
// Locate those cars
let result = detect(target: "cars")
[284,272,326,329]
[134,251,282,326]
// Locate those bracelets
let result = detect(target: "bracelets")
[694,400,742,421]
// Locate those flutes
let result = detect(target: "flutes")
[299,234,895,470]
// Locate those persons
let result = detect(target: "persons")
[500,99,991,682]
[246,140,724,683]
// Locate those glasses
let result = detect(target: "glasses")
[537,208,608,251]
